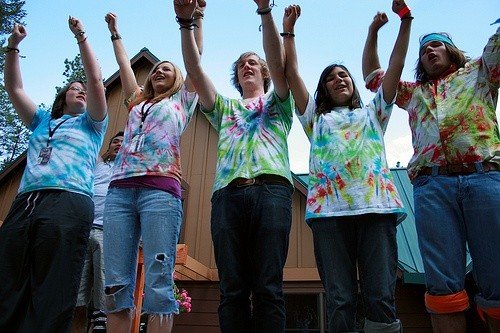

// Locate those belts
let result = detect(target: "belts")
[417,161,498,176]
[224,175,291,188]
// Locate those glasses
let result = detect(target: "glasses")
[67,85,87,94]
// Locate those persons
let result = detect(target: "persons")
[173,0,295,333]
[0,16,109,333]
[362,11,500,333]
[70,131,127,333]
[103,0,208,333]
[282,0,415,333]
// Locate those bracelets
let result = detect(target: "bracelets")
[280,32,295,38]
[110,33,122,41]
[5,46,20,53]
[256,0,278,15]
[77,36,88,44]
[398,6,410,18]
[74,31,85,38]
[401,16,414,21]
[174,7,204,30]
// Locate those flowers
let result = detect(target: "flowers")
[142,276,191,312]
